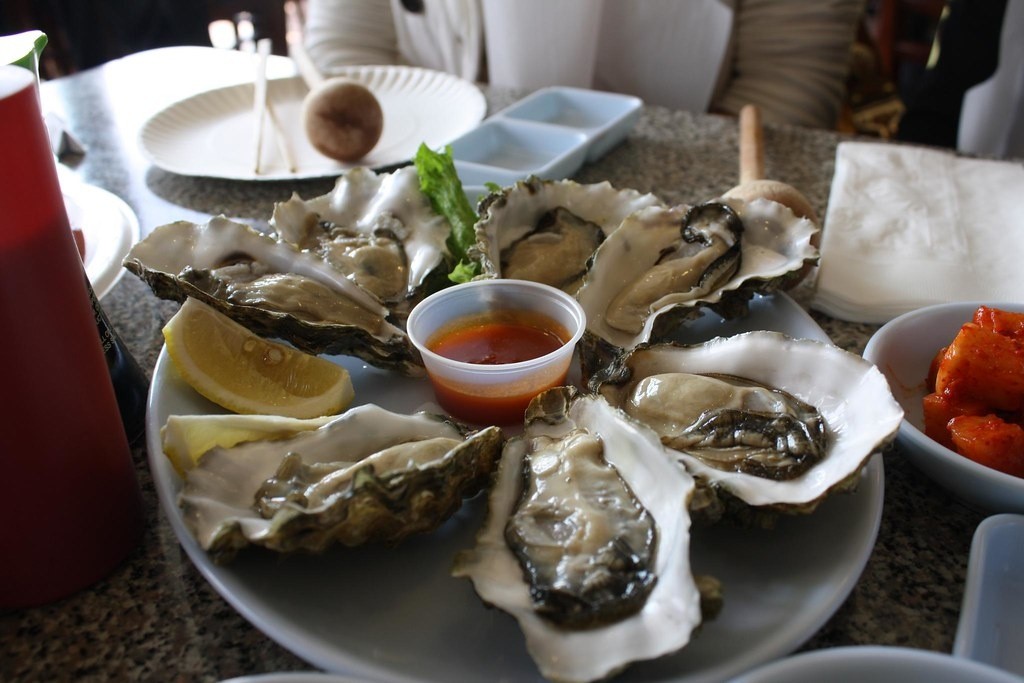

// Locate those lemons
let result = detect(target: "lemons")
[161,294,356,420]
[161,411,342,483]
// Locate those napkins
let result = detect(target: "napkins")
[812,140,1024,324]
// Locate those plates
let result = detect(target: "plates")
[61,184,140,301]
[431,84,645,209]
[146,186,883,683]
[730,645,1024,683]
[138,65,489,181]
[953,514,1024,678]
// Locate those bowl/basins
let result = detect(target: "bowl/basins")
[406,277,587,429]
[863,300,1024,512]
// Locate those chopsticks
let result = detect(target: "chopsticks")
[236,19,297,175]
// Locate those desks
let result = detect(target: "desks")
[1,47,1024,683]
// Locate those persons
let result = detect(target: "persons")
[285,0,858,129]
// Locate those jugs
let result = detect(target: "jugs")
[0,30,149,445]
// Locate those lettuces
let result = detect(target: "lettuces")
[415,140,503,292]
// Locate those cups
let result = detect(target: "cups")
[0,63,145,611]
